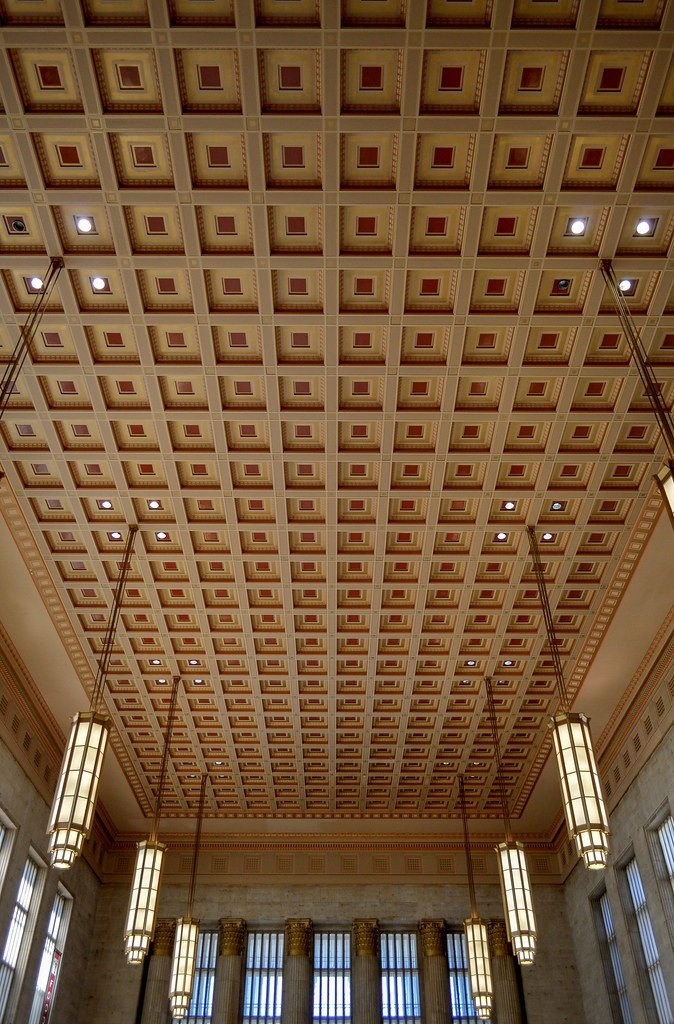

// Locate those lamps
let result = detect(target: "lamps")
[525,525,610,870]
[123,675,182,964]
[456,773,494,1020]
[44,523,140,869]
[166,773,207,1019]
[597,258,674,529]
[482,675,539,965]
